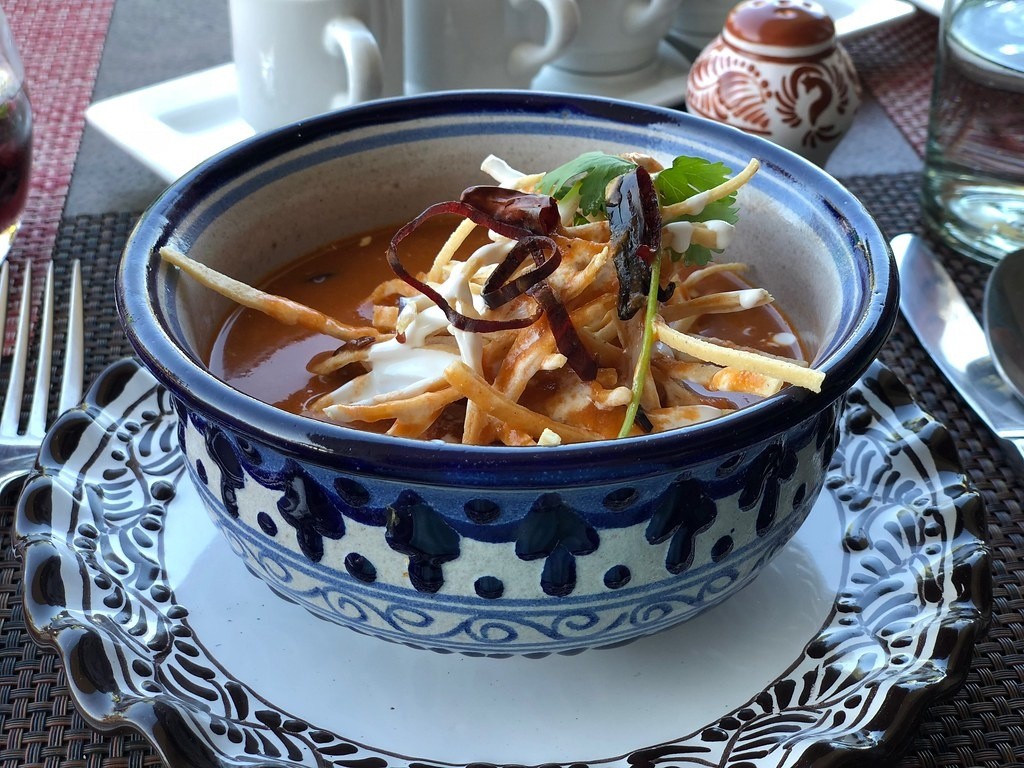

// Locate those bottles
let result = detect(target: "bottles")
[923,0,1023,266]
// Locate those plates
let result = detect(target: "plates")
[12,357,995,768]
[85,37,695,184]
[669,0,918,52]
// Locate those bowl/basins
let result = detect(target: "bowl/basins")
[984,248,1024,406]
[113,90,901,656]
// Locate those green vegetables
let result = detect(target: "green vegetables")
[534,151,742,440]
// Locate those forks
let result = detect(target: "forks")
[0,252,85,492]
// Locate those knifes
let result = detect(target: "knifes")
[889,231,1024,484]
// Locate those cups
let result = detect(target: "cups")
[541,1,680,76]
[403,1,582,92]
[228,1,404,136]
[0,8,34,269]
